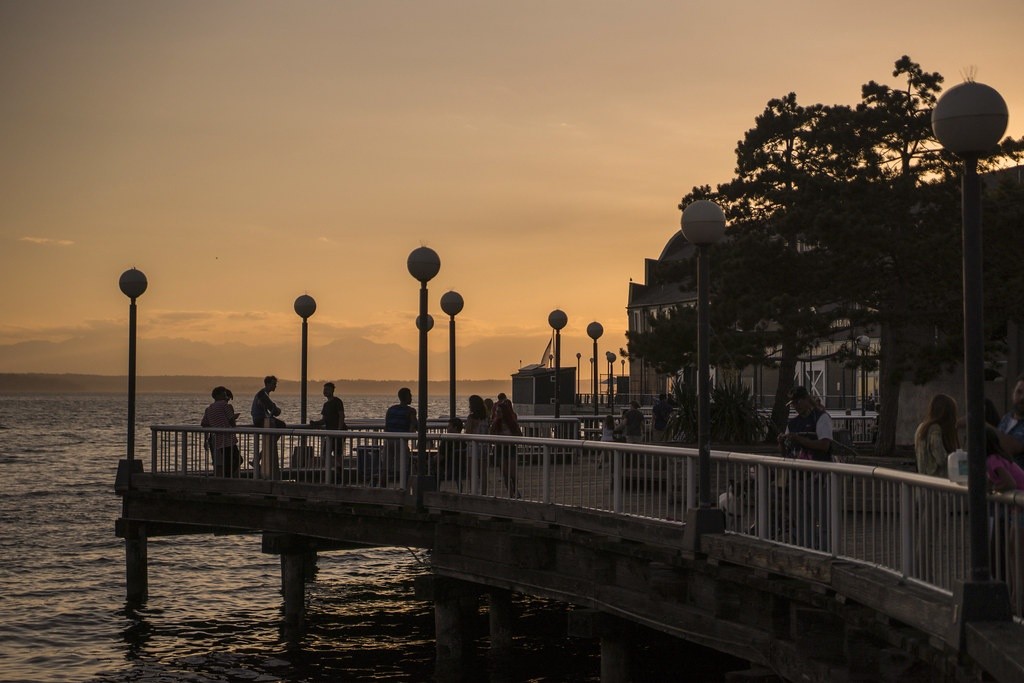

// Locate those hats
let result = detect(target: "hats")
[785,384,810,407]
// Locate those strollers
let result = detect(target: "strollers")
[599,430,626,469]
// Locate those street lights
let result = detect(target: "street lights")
[405,247,439,515]
[295,295,316,468]
[115,269,148,483]
[441,291,464,417]
[931,82,1013,622]
[681,199,725,534]
[607,353,616,415]
[606,351,611,394]
[857,336,871,416]
[621,360,625,376]
[548,310,567,437]
[576,353,581,394]
[589,358,594,397]
[587,322,604,436]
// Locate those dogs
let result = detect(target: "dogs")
[717,484,747,531]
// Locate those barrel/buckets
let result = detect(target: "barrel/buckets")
[947,449,968,482]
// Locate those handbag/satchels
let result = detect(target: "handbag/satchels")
[822,437,858,483]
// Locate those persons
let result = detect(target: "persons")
[383,387,525,499]
[310,382,348,482]
[200,386,243,478]
[250,376,285,467]
[598,391,675,467]
[909,378,1024,611]
[777,387,835,553]
